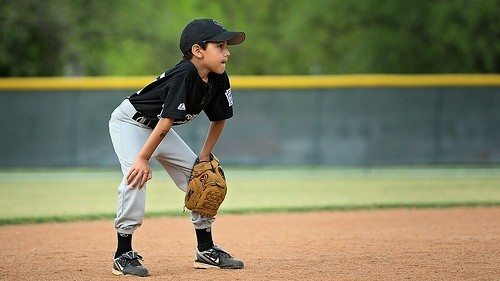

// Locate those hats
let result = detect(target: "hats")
[179,19,245,54]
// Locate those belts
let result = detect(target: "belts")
[133,112,158,128]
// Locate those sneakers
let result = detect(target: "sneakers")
[111,250,148,277]
[193,246,243,268]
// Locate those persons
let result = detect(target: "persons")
[109,18,246,277]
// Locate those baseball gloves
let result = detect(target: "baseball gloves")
[184,153,228,218]
